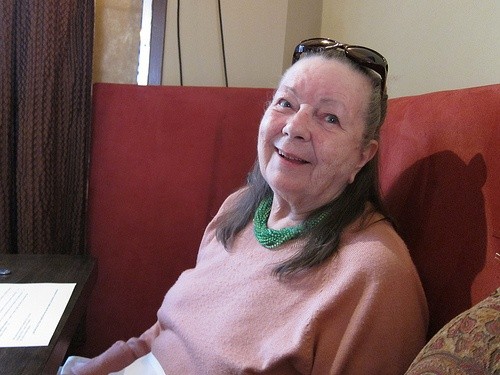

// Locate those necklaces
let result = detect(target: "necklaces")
[253,193,338,248]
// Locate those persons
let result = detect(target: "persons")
[58,37,429,373]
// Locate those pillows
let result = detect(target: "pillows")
[404,285,500,375]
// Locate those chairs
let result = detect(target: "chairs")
[90,82,500,375]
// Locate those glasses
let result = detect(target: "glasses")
[292,37,389,130]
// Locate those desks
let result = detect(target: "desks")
[0,252,100,374]
